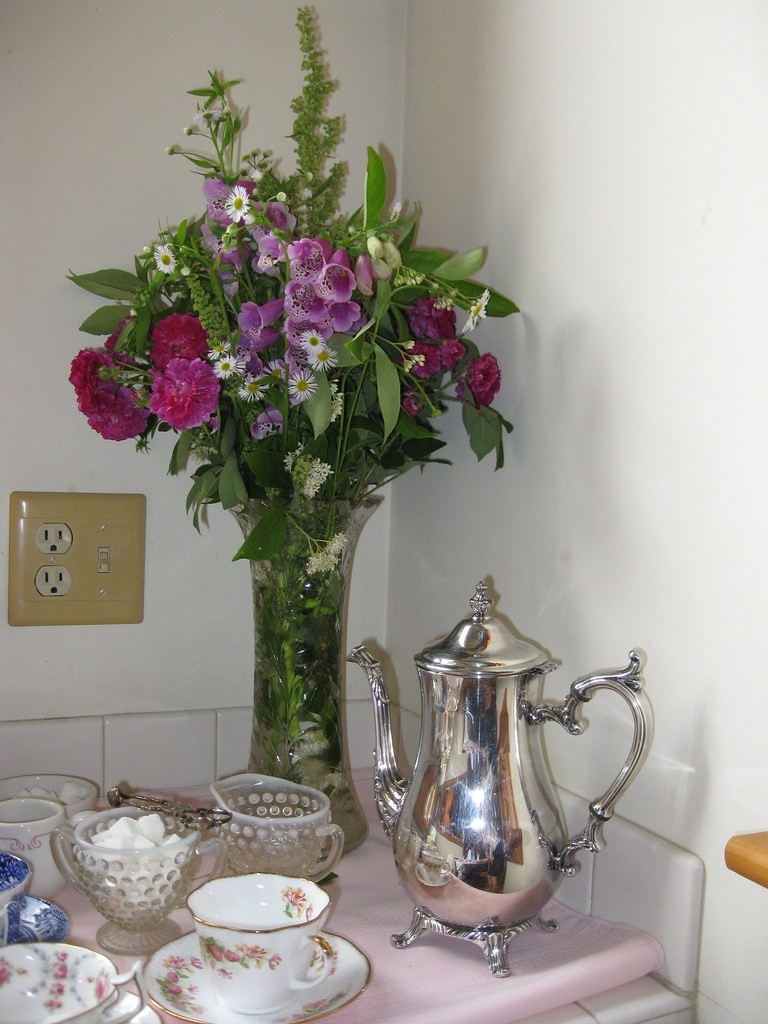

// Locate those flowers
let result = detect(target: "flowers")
[69,8,524,799]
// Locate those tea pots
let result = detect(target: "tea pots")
[346,582,655,973]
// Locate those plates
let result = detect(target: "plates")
[0,894,69,946]
[142,928,372,1024]
[112,989,163,1024]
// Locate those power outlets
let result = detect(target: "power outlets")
[9,492,145,625]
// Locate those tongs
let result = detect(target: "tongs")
[106,786,233,832]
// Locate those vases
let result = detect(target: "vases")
[226,495,386,855]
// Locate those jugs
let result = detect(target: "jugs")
[209,773,344,884]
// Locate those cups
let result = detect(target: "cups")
[0,798,98,902]
[49,806,227,956]
[0,852,30,945]
[0,773,100,818]
[186,872,337,1014]
[0,941,148,1024]
